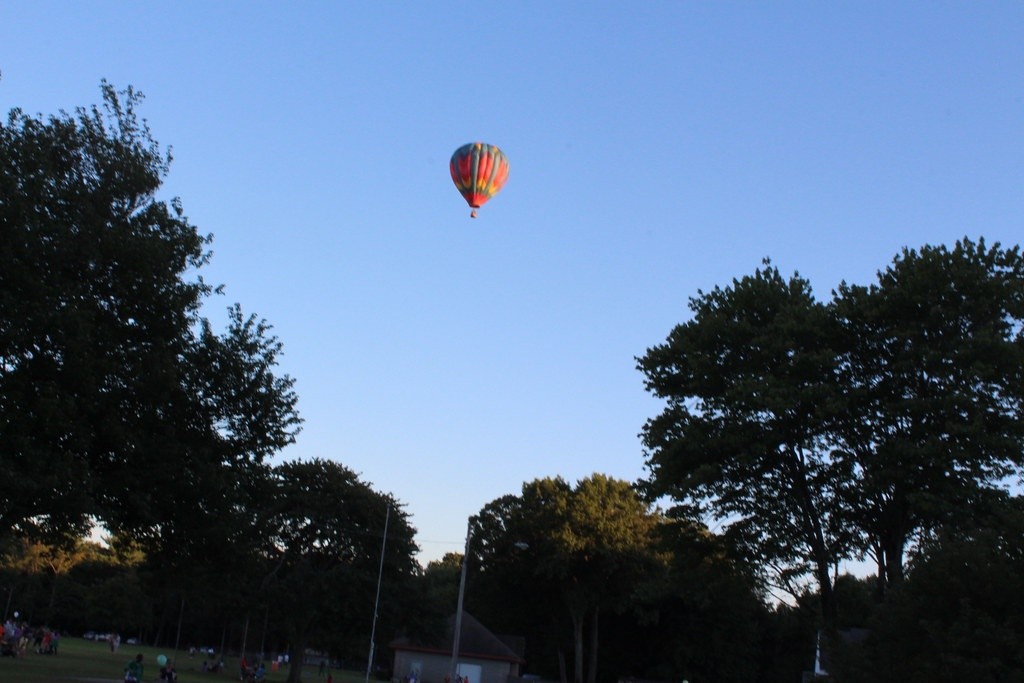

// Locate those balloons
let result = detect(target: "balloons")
[156,654,167,667]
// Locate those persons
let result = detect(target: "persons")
[189,644,225,674]
[160,658,178,683]
[277,652,289,672]
[408,668,421,683]
[109,631,120,655]
[444,671,469,683]
[240,654,265,683]
[318,659,326,678]
[123,653,144,683]
[0,619,61,659]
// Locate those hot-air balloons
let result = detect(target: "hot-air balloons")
[449,142,511,218]
